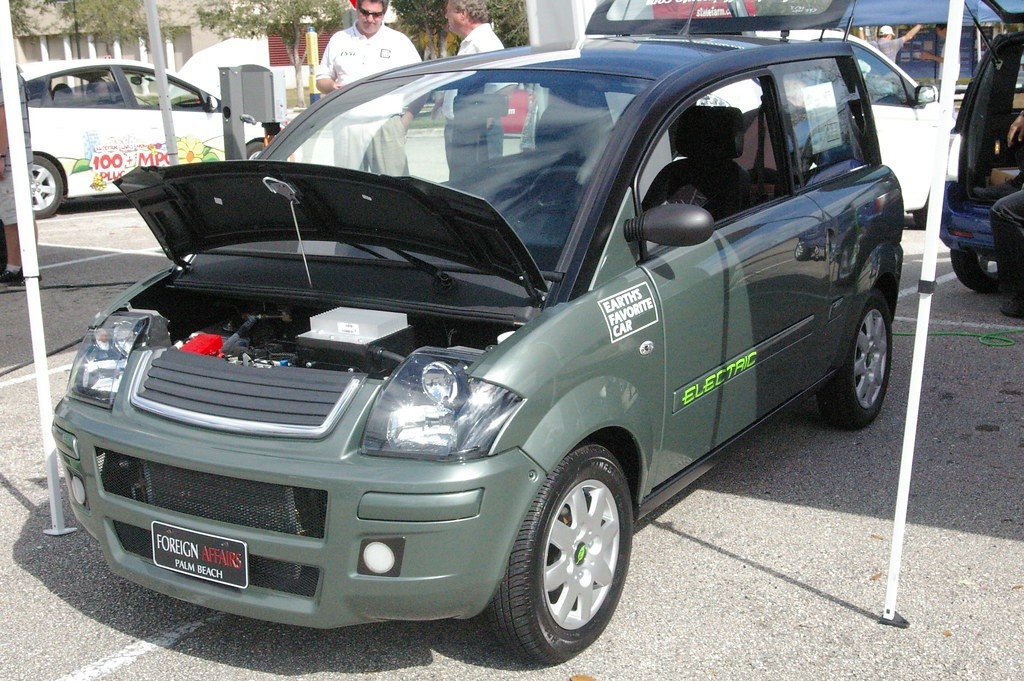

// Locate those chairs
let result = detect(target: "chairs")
[643,106,752,220]
[86,77,118,105]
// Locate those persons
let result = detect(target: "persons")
[858,23,921,64]
[972,109,1024,318]
[921,24,961,101]
[316,0,430,177]
[85,77,110,106]
[430,0,518,181]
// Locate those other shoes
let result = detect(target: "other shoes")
[0,266,22,283]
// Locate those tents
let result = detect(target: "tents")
[839,0,1024,65]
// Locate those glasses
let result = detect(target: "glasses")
[358,6,384,17]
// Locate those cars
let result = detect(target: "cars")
[431,0,1024,307]
[52,0,902,670]
[14,37,299,220]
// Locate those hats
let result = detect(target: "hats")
[878,25,896,37]
